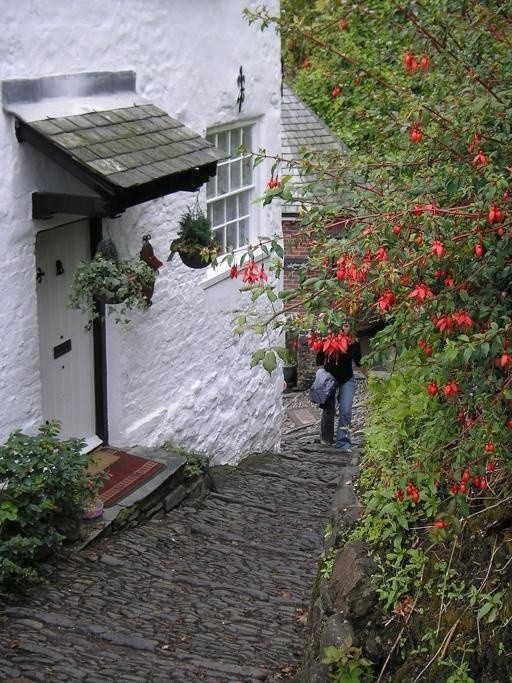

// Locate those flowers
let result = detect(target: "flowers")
[84,467,113,501]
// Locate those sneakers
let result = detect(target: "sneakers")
[320,438,332,447]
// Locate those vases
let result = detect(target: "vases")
[83,499,104,520]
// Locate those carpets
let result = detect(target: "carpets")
[85,445,167,508]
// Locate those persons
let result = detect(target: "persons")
[314,318,371,451]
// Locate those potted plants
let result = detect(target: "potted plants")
[170,205,222,269]
[283,354,297,382]
[67,242,157,332]
[0,419,88,544]
